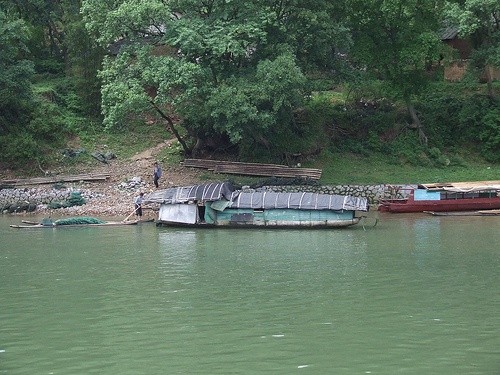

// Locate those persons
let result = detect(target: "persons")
[134,191,145,221]
[152,162,161,191]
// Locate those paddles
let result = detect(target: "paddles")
[121,205,140,223]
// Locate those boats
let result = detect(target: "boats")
[423,210,499,217]
[378,183,500,213]
[11,215,154,229]
[143,181,368,231]
